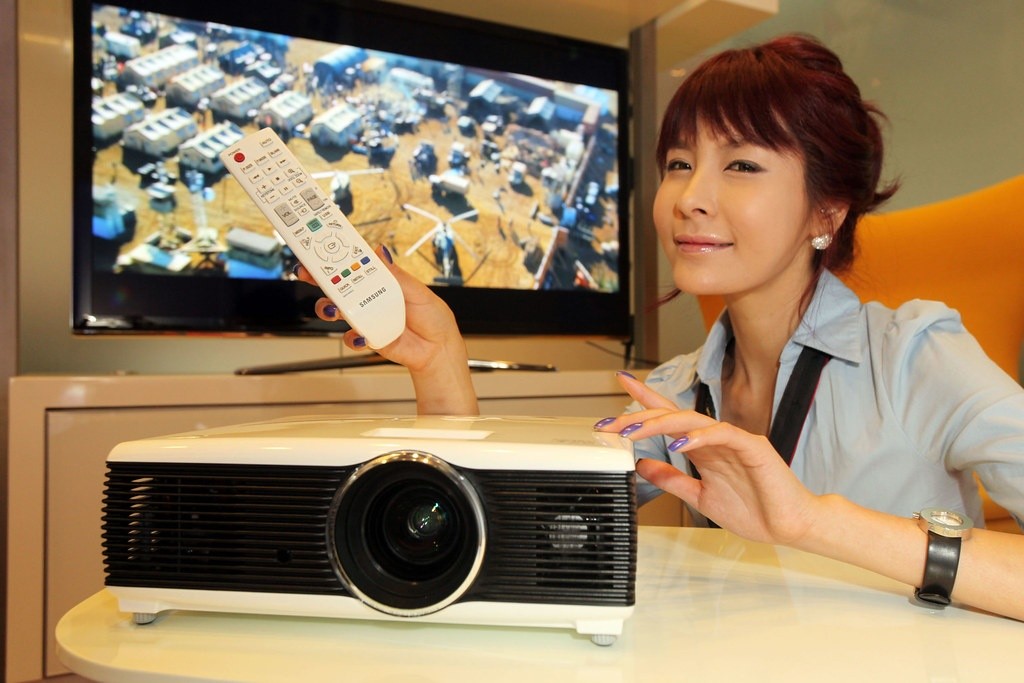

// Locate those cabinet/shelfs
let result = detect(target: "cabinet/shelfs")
[5,371,707,683]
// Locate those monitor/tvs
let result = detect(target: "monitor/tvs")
[69,0,630,376]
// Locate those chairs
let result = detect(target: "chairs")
[700,172,1024,537]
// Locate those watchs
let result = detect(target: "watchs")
[914,506,975,608]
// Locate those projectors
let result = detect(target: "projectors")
[102,412,638,647]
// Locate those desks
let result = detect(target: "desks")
[56,526,1024,683]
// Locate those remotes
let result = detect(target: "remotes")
[218,126,406,349]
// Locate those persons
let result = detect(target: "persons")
[292,31,1024,622]
